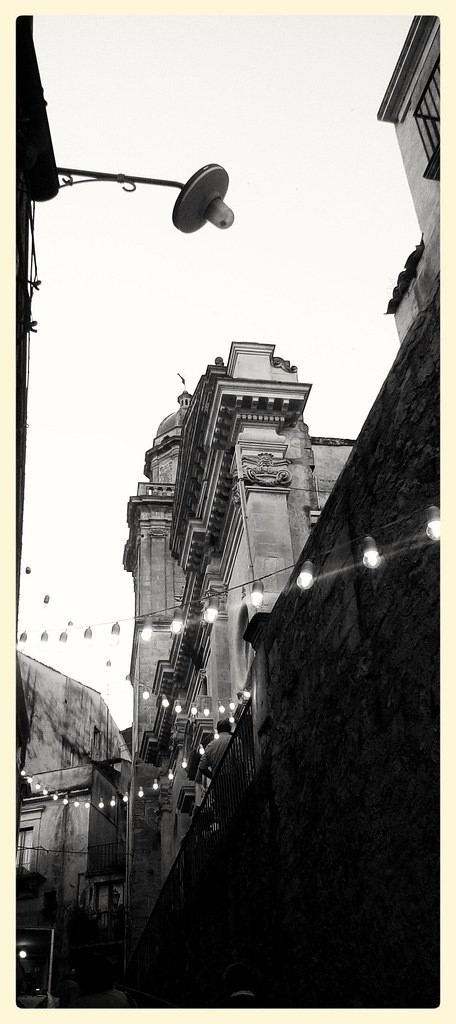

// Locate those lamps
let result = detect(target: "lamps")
[57,164,235,234]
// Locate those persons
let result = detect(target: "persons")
[17,957,130,1008]
[221,962,267,1007]
[198,720,246,831]
